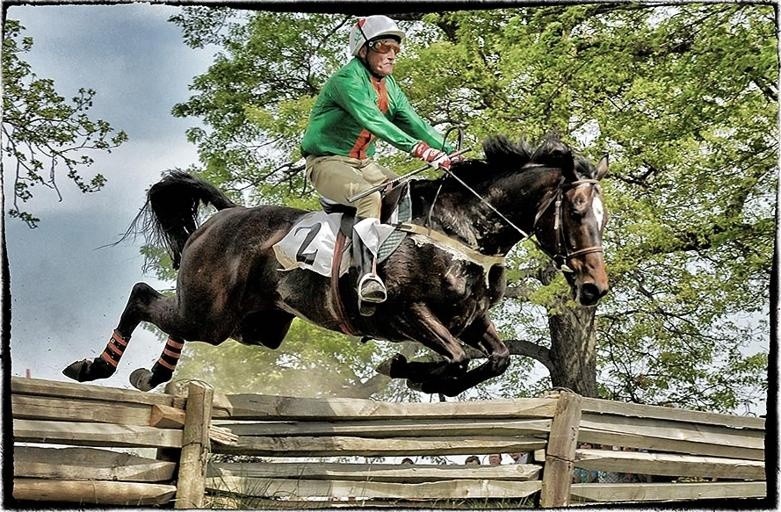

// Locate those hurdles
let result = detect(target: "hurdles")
[11,377,767,509]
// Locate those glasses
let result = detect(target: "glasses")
[373,42,401,53]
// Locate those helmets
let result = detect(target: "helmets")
[343,16,404,53]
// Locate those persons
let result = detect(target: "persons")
[300,12,464,320]
[398,436,682,485]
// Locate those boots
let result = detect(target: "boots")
[354,217,385,315]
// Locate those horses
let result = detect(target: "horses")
[62,135,611,397]
